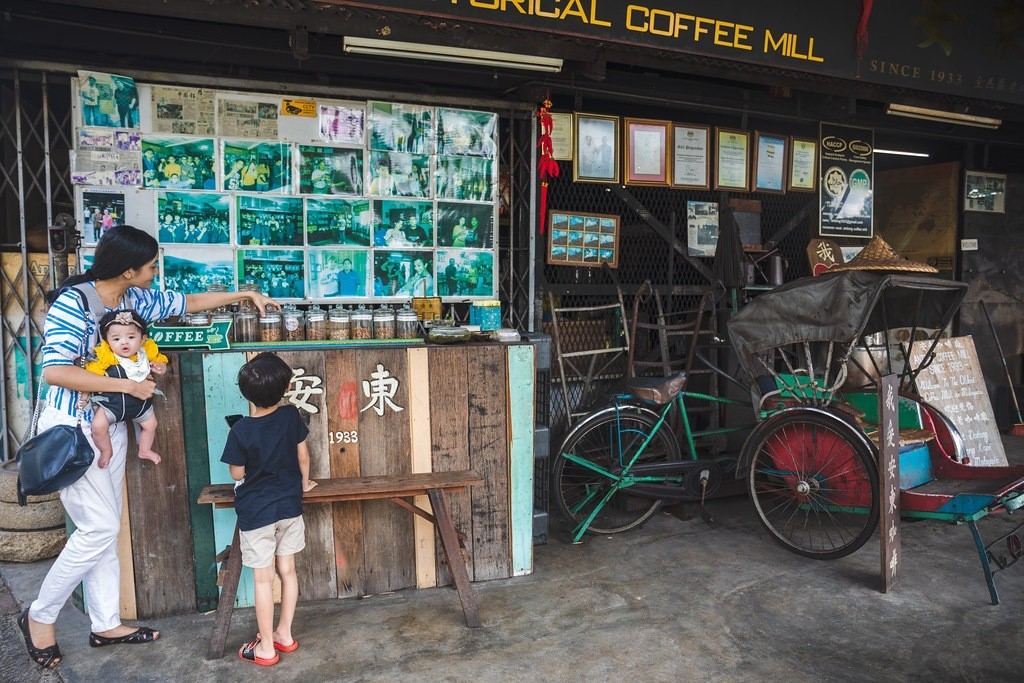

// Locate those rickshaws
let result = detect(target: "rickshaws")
[726,266,1024,605]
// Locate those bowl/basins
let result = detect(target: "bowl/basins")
[428,328,469,343]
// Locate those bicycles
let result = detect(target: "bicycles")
[548,371,884,561]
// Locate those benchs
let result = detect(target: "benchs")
[195,469,484,655]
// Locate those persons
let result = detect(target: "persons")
[329,214,346,244]
[143,148,215,190]
[384,214,428,247]
[312,159,346,194]
[442,175,484,200]
[221,351,318,665]
[16,225,282,669]
[445,258,478,296]
[244,269,304,298]
[371,159,423,195]
[75,308,169,469]
[163,273,233,294]
[452,216,480,247]
[225,159,270,191]
[84,207,112,241]
[83,76,136,127]
[374,255,433,296]
[159,208,229,244]
[251,215,295,246]
[319,256,360,296]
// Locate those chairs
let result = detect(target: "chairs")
[749,370,938,492]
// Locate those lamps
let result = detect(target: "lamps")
[343,37,563,74]
[887,104,1002,129]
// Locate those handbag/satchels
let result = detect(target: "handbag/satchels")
[13,424,94,507]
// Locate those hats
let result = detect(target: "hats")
[817,233,940,274]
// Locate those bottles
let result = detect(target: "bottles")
[179,303,418,344]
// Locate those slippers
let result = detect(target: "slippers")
[239,640,280,666]
[256,629,298,653]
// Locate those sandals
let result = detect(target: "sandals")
[17,606,62,669]
[89,621,160,647]
